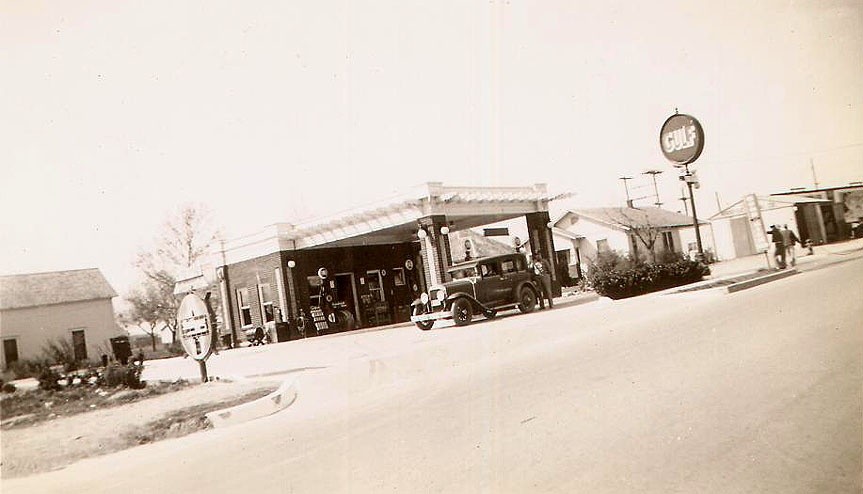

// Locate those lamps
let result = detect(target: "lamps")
[286,255,296,268]
[546,218,554,229]
[440,223,449,235]
[417,222,428,239]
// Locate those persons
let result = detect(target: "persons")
[766,223,801,269]
[526,250,556,309]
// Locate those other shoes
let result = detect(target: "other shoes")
[539,307,545,310]
[550,306,553,308]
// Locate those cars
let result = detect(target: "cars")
[408,251,546,331]
